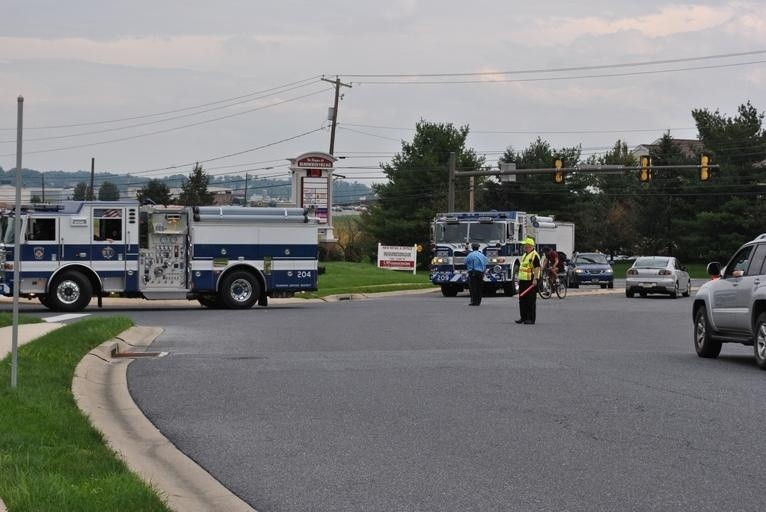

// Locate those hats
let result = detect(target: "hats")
[519,238,534,246]
[472,243,480,248]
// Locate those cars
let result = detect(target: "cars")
[570,251,617,292]
[625,256,695,299]
[606,255,640,261]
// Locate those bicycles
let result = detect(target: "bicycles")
[535,267,569,302]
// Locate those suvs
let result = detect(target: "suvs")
[690,233,765,367]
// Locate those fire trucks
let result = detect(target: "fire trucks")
[1,197,323,312]
[428,208,576,296]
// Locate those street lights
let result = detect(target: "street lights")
[243,166,274,208]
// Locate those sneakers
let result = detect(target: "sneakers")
[515,318,535,324]
[469,302,481,306]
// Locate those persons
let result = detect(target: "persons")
[542,246,562,295]
[514,238,541,324]
[464,244,489,306]
[732,270,744,277]
